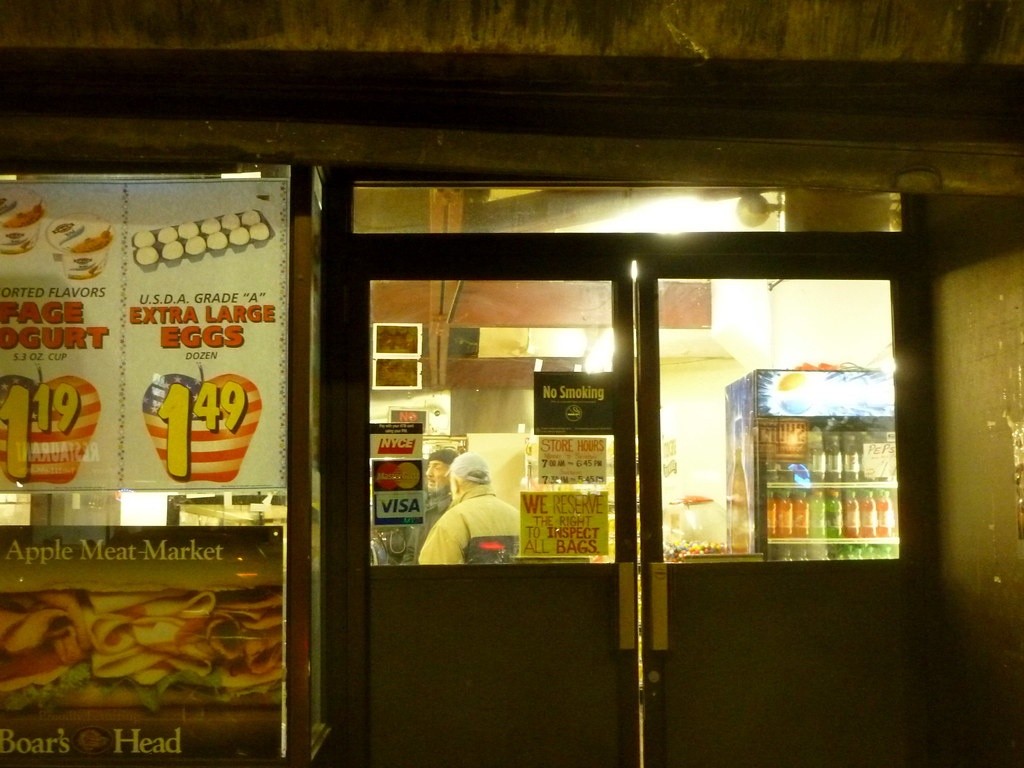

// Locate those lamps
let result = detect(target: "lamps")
[736,188,901,232]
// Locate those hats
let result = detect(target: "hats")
[445,452,491,484]
[429,449,459,465]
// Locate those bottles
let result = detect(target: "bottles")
[808,427,877,483]
[768,544,899,560]
[728,418,751,555]
[767,489,896,538]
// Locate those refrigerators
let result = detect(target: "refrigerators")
[724,367,898,564]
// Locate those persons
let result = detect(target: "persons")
[400,450,455,564]
[419,452,522,566]
